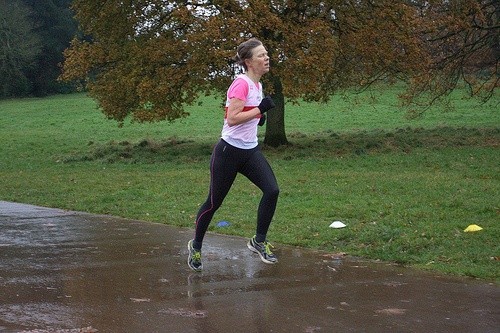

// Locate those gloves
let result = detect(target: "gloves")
[258,95,275,114]
[258,114,266,126]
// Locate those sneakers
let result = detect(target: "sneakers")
[187,239,203,272]
[247,235,278,265]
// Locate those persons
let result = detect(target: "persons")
[188,38,279,272]
